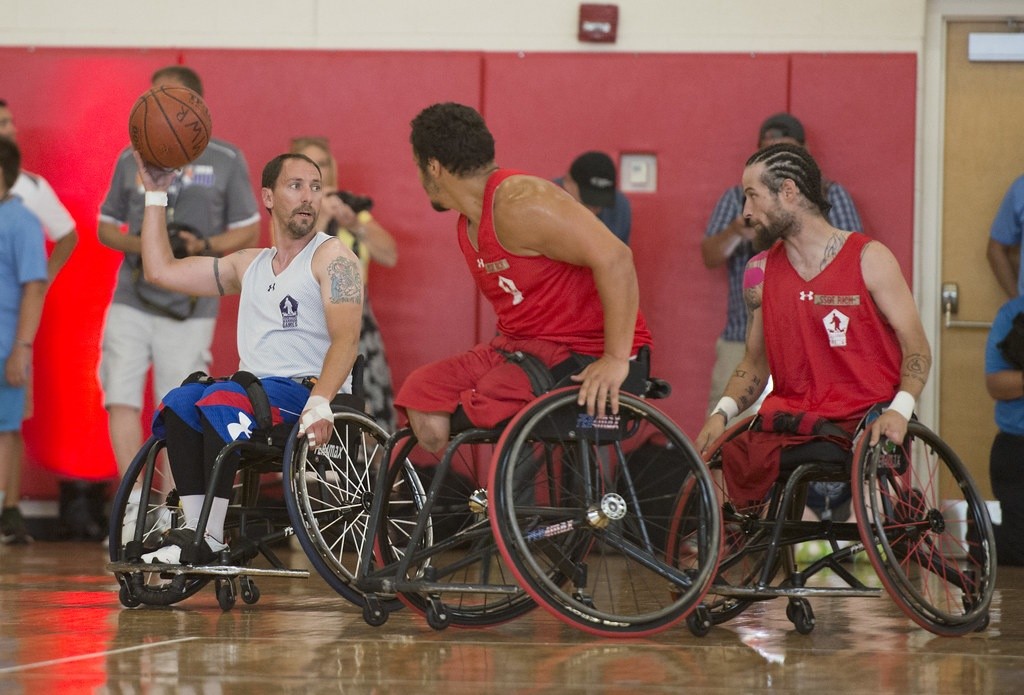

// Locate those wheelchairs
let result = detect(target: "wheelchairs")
[349,343,727,638]
[666,387,999,640]
[107,353,436,617]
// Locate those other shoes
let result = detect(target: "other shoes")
[0,506,26,544]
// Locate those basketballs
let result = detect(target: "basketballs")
[127,85,212,170]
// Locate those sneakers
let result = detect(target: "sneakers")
[141,527,231,566]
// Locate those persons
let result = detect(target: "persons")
[552,150,631,246]
[96,65,263,549]
[0,67,76,547]
[678,114,932,559]
[983,174,1024,568]
[128,136,400,563]
[393,101,656,454]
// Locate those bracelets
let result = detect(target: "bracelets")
[14,339,34,348]
[888,391,916,423]
[145,191,169,207]
[711,396,739,425]
[302,396,330,412]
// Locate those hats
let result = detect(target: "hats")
[570,151,617,209]
[760,114,805,145]
